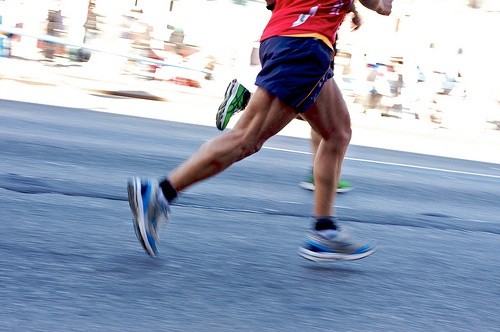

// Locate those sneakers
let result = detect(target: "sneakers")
[299,165,354,195]
[126,174,172,259]
[297,227,380,263]
[215,80,249,131]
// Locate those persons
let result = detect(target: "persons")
[215,0,354,193]
[127,0,393,263]
